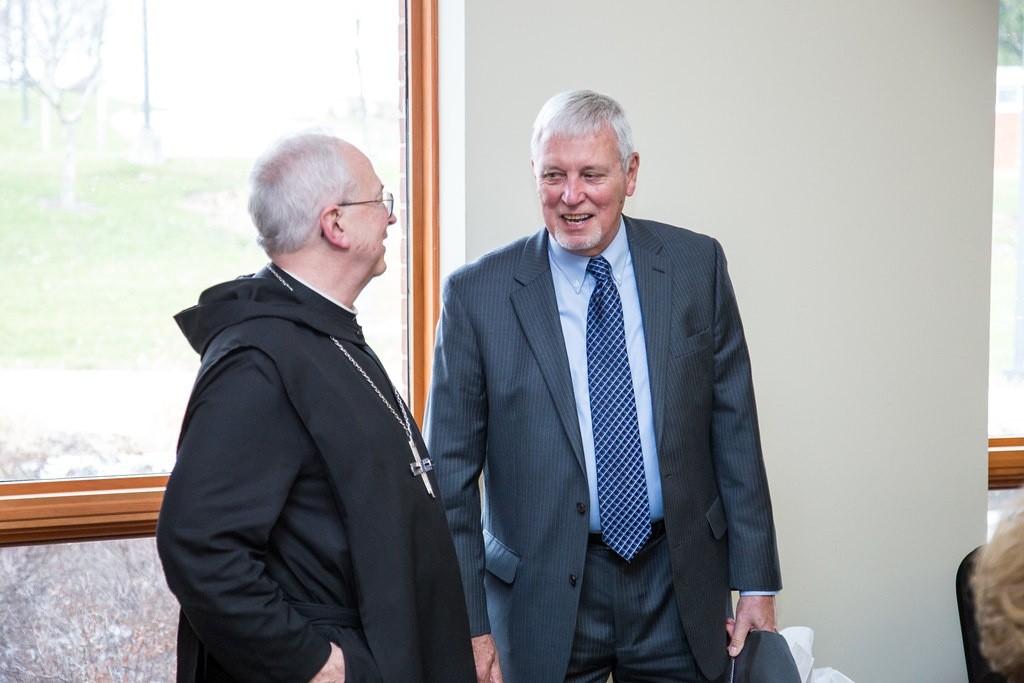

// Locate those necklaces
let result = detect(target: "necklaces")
[266,265,435,497]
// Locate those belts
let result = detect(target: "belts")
[588,519,665,549]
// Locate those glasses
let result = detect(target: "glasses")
[337,193,395,219]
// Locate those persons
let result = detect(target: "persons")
[156,134,477,683]
[420,90,782,683]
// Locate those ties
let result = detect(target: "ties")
[586,255,653,564]
